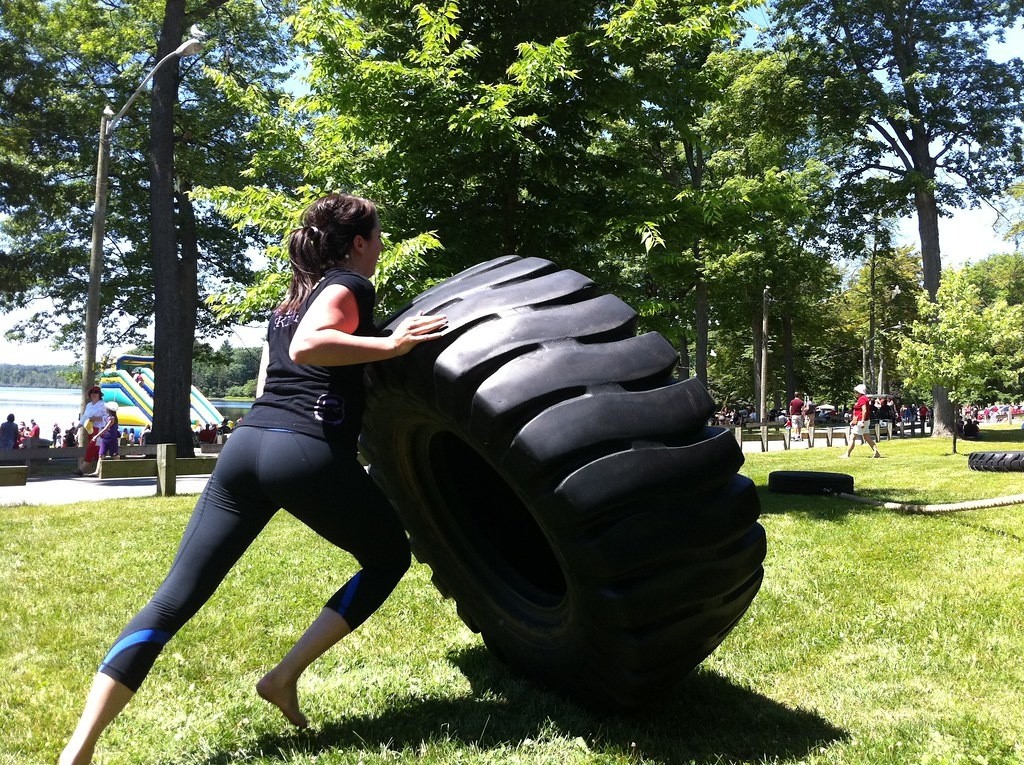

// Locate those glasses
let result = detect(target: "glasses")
[90,390,99,394]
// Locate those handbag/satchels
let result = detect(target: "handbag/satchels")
[801,401,815,415]
[96,416,106,446]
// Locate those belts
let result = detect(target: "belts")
[792,413,801,415]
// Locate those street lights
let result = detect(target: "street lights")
[81,37,206,404]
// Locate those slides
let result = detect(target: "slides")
[99,366,229,446]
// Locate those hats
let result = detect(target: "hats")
[7,414,14,422]
[88,386,104,396]
[84,419,93,434]
[854,384,866,394]
[105,402,118,411]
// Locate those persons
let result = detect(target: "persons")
[711,384,1024,459]
[0,374,243,478]
[58,192,450,765]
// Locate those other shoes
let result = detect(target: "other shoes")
[73,468,83,476]
[874,450,880,457]
